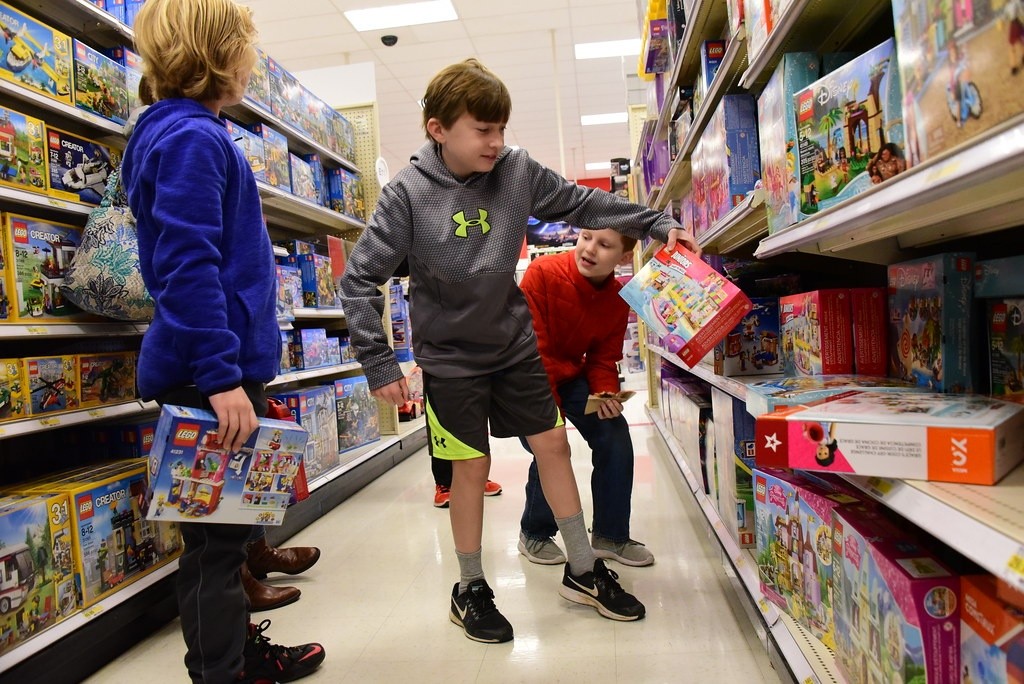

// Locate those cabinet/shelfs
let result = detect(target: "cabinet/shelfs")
[1,1,433,684]
[637,1,1024,684]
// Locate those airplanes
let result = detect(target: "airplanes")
[61,154,108,197]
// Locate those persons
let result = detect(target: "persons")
[422,372,503,510]
[121,0,328,684]
[335,59,703,644]
[515,226,655,568]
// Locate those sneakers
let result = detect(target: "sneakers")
[590,529,655,566]
[517,528,566,564]
[434,483,451,508]
[559,558,645,621]
[449,579,514,644]
[484,479,502,496]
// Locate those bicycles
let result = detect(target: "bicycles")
[946,60,984,121]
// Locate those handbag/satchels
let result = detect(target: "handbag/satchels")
[60,160,164,322]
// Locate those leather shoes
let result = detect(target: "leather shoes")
[237,670,282,684]
[240,561,302,613]
[244,623,325,683]
[246,535,321,580]
